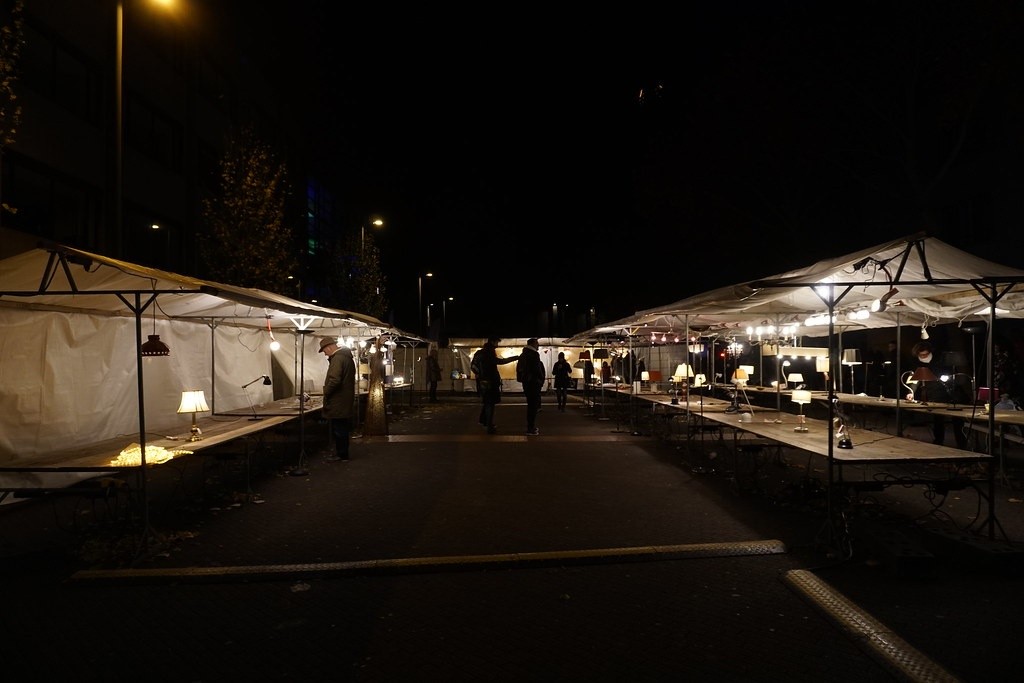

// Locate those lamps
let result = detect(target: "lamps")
[977,387,1000,415]
[901,367,973,411]
[140,278,170,356]
[579,299,893,449]
[241,375,271,421]
[920,311,930,340]
[264,308,280,351]
[177,390,211,442]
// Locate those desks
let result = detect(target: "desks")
[587,384,1010,561]
[452,378,597,395]
[0,383,413,558]
[714,383,1024,489]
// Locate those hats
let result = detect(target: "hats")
[319,336,337,352]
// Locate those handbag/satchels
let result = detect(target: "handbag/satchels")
[565,377,571,388]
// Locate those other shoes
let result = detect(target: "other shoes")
[526,428,539,436]
[327,455,348,462]
[482,423,496,427]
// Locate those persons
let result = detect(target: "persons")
[600,350,645,384]
[470,336,519,435]
[319,336,356,463]
[583,361,595,394]
[425,350,443,403]
[552,352,572,412]
[993,342,1017,395]
[516,338,545,435]
[868,340,907,397]
[906,342,969,448]
[360,343,372,379]
